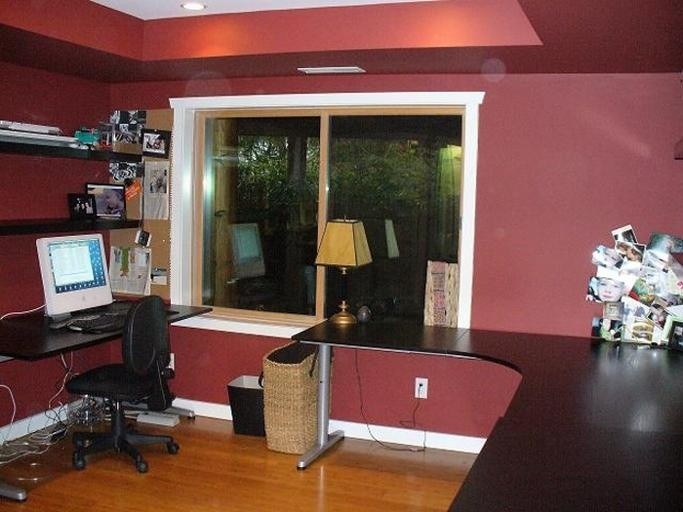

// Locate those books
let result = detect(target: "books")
[109,247,151,296]
[0,120,89,150]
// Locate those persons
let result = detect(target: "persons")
[596,277,625,303]
[74,198,93,214]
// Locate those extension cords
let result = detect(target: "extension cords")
[137,410,181,428]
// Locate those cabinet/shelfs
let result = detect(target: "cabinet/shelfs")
[0,135,142,234]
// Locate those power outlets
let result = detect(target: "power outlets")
[414,377,428,399]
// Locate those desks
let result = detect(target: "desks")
[0,306,212,502]
[291,315,683,512]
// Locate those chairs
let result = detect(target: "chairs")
[64,296,175,473]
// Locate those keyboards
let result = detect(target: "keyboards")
[66,310,128,334]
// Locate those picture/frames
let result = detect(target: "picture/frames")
[86,184,126,219]
[668,321,683,352]
[141,128,172,159]
[67,193,96,219]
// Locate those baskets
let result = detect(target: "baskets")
[263,341,334,454]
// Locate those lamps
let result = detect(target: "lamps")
[315,218,373,326]
[358,217,401,313]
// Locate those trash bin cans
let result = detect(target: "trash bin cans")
[227,374,266,437]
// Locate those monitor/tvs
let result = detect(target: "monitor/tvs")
[231,222,267,281]
[35,232,113,330]
